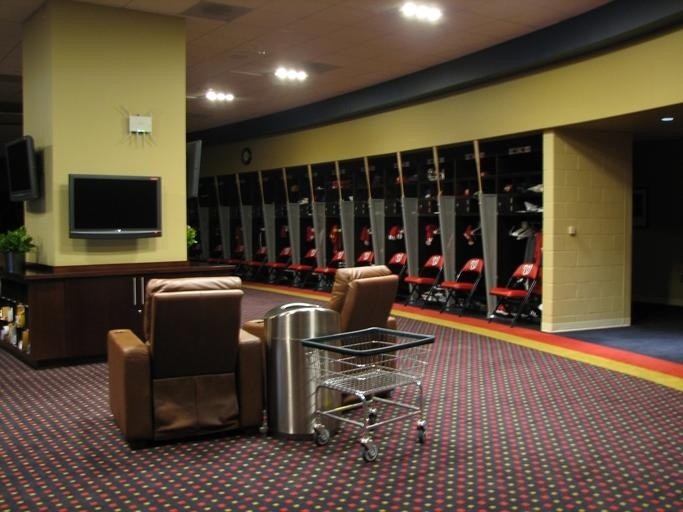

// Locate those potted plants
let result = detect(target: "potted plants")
[0,224,37,273]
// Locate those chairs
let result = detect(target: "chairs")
[405,254,445,310]
[483,263,541,330]
[439,258,484,314]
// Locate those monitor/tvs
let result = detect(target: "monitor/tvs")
[68,174,162,240]
[186,139,203,198]
[4,135,40,201]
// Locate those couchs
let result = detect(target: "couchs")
[105,275,267,443]
[238,265,400,412]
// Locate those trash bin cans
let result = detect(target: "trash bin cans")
[263,303,344,441]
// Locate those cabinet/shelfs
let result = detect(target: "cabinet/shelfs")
[197,124,542,324]
[0,262,232,368]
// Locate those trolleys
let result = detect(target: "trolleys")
[300,326,436,463]
